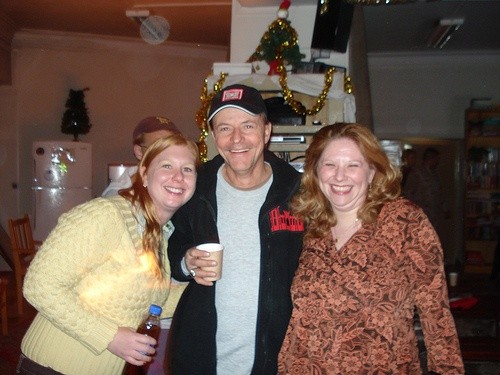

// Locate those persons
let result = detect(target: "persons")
[15,116,200,375]
[167,84,306,375]
[277,122,465,375]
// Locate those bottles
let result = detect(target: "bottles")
[121,304,162,375]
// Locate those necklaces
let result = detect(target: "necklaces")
[333,218,359,242]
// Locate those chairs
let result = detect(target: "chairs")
[9,213,46,322]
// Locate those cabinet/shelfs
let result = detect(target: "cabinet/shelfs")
[463,108,500,276]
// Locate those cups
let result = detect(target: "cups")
[196,243,224,281]
[449,272,458,287]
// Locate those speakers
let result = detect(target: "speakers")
[311,0,354,53]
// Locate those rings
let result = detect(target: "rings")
[190,268,200,276]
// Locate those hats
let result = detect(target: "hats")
[206,84,266,121]
[133,117,178,143]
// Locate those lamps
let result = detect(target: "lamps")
[428,19,464,49]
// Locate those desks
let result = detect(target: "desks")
[415,274,500,361]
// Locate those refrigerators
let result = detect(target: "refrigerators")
[33,142,93,242]
